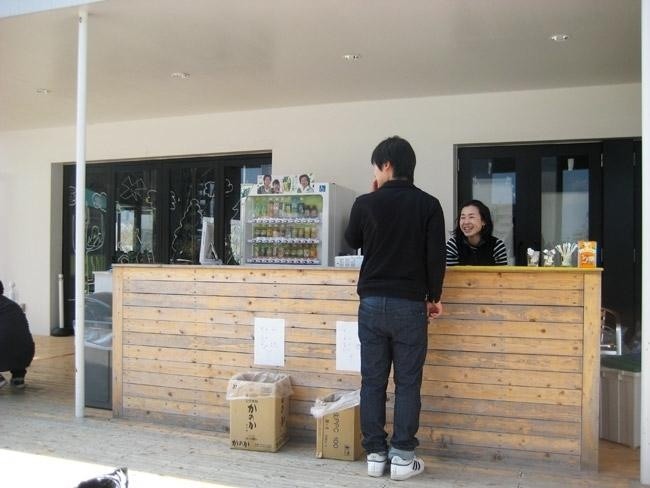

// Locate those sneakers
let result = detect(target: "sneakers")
[9,377,25,389]
[367,451,387,476]
[0,375,6,388]
[390,456,425,481]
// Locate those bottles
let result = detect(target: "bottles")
[250,196,320,261]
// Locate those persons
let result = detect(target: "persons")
[446,200,507,266]
[344,135,446,480]
[258,175,272,193]
[0,280,35,389]
[297,175,314,194]
[272,180,280,194]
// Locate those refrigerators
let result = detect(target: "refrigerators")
[239,181,355,269]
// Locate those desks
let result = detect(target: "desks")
[112,264,603,473]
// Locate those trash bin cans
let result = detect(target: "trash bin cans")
[72,290,113,411]
[314,392,367,462]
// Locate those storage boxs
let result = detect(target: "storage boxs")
[314,392,361,459]
[599,353,641,449]
